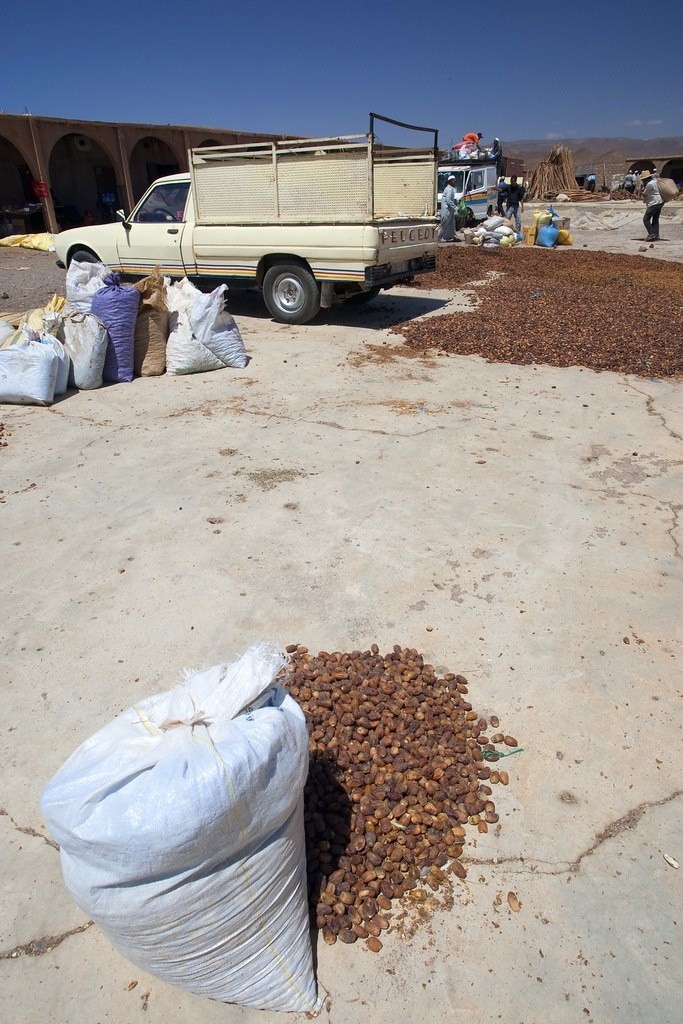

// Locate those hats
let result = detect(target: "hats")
[635,171,637,173]
[638,170,653,181]
[653,169,657,171]
[629,170,633,173]
[478,133,483,138]
[448,175,456,181]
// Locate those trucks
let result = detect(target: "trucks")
[437,158,499,230]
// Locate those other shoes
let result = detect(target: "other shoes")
[646,233,656,241]
[454,238,461,242]
[656,236,660,240]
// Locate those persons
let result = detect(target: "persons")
[494,175,525,231]
[623,169,659,194]
[497,176,506,218]
[638,170,665,241]
[491,138,502,174]
[438,175,462,243]
[463,132,483,150]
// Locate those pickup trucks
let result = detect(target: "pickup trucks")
[53,111,441,325]
